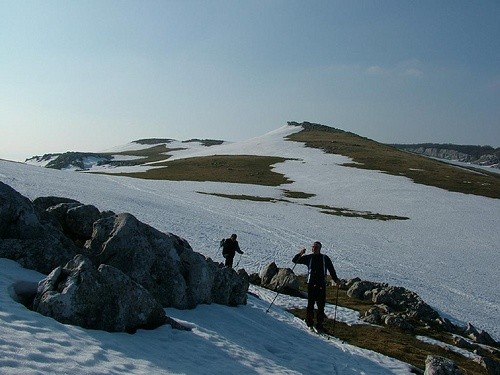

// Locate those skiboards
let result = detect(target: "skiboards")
[312,327,349,346]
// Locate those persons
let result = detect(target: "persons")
[220,234,245,270]
[291,241,342,333]
